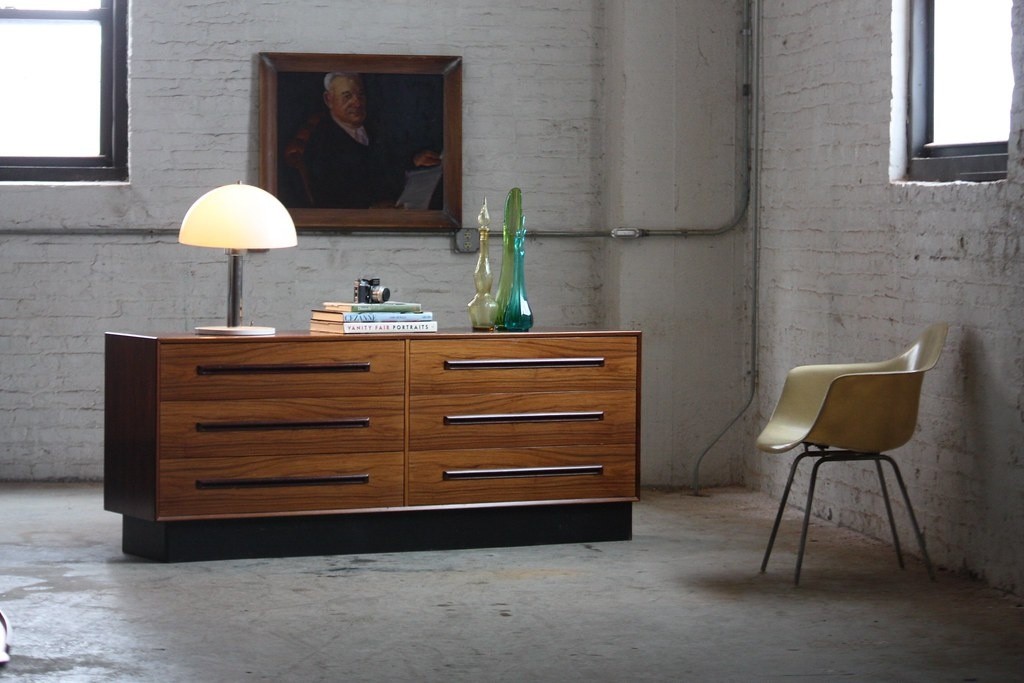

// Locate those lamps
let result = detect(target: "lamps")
[178,180,298,336]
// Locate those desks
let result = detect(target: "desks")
[103,329,642,563]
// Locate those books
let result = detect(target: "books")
[309,301,438,335]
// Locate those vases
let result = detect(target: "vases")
[491,187,534,333]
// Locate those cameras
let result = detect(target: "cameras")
[354,278,390,304]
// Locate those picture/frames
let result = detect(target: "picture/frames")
[257,52,462,230]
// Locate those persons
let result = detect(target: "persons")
[304,72,442,210]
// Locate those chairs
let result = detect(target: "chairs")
[755,320,948,589]
